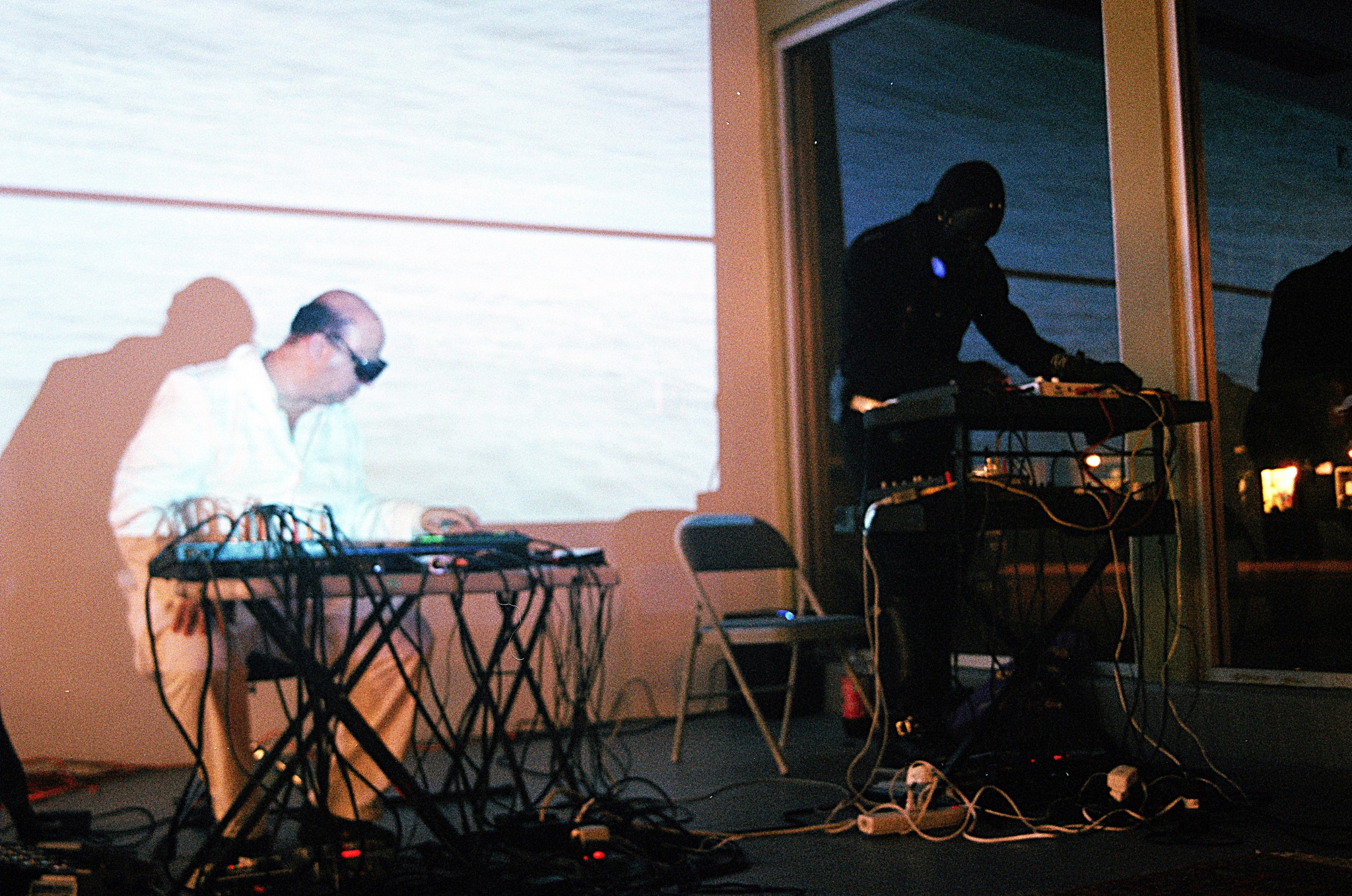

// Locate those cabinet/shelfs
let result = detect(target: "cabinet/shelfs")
[158,563,620,896]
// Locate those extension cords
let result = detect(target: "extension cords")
[857,804,968,836]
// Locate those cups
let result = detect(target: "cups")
[842,673,874,737]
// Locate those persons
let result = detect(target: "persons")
[108,288,480,867]
[844,161,1147,768]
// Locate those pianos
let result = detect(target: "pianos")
[863,376,1213,445]
[829,462,1184,812]
[149,531,609,896]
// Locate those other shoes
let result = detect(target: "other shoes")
[879,706,962,776]
[298,799,399,852]
[217,828,278,870]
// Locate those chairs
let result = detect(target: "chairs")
[667,512,887,774]
[173,653,332,827]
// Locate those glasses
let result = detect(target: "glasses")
[318,326,386,383]
